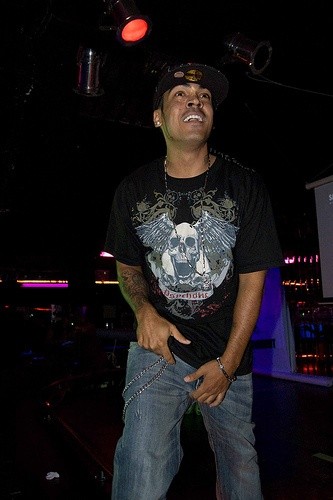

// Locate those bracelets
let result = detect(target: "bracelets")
[216,356,238,383]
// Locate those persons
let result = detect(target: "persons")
[91,59,280,500]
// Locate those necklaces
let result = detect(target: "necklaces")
[163,152,212,291]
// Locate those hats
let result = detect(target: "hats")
[153,63,230,111]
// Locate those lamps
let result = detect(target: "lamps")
[102,0,151,47]
[71,46,104,98]
[222,34,272,79]
